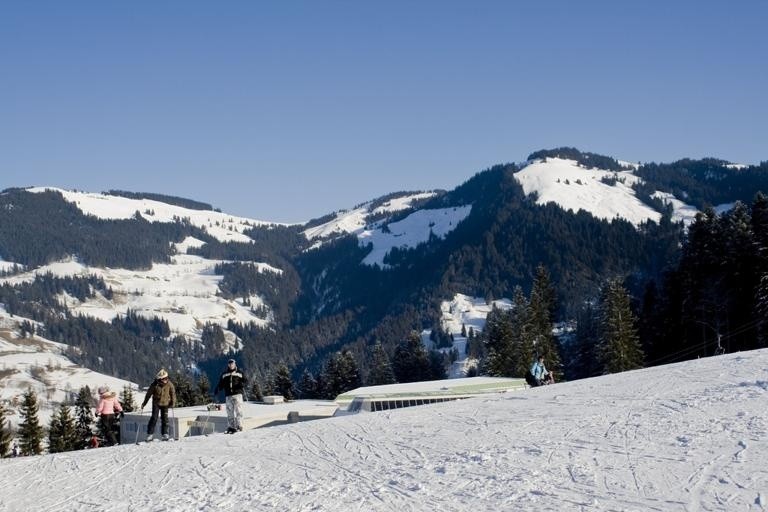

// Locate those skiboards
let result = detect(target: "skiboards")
[136,438,178,446]
[224,427,243,435]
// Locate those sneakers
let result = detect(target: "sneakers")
[224,426,243,434]
[162,433,169,441]
[144,433,154,442]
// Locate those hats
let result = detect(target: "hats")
[155,368,169,379]
[98,385,110,394]
[228,359,236,364]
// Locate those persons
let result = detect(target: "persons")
[547,367,556,384]
[531,355,549,385]
[95,386,125,446]
[91,436,99,449]
[214,358,247,434]
[141,369,177,442]
[13,445,20,457]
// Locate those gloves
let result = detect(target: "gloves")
[95,412,98,417]
[120,411,125,418]
